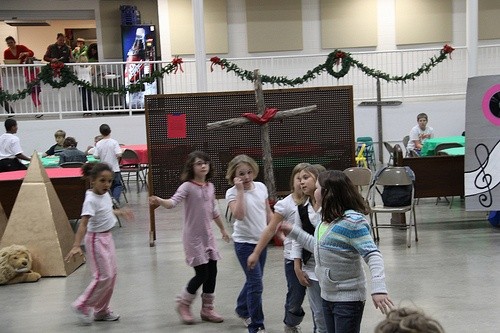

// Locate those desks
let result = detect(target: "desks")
[19,151,100,167]
[421,133,465,155]
[397,155,463,198]
[0,167,84,220]
[118,144,150,183]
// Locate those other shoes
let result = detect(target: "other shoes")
[284,325,302,333]
[112,198,121,208]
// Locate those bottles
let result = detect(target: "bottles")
[125,28,155,82]
[392,144,404,167]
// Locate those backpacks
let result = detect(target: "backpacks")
[375,167,411,206]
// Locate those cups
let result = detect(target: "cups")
[75,47,80,54]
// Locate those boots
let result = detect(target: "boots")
[175,290,197,324]
[200,293,225,322]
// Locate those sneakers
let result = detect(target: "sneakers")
[256,326,265,333]
[94,311,120,320]
[73,306,92,325]
[242,314,252,327]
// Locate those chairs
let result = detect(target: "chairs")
[344,136,463,248]
[120,149,147,194]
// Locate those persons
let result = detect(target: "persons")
[148,149,231,326]
[225,154,280,333]
[71,37,99,119]
[59,137,89,168]
[3,36,44,118]
[41,33,71,64]
[84,135,103,155]
[276,170,394,333]
[40,129,66,158]
[0,118,33,172]
[406,113,435,157]
[94,124,124,211]
[374,306,444,333]
[66,160,121,323]
[247,161,325,333]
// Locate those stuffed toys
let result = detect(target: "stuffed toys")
[0,245,40,286]
